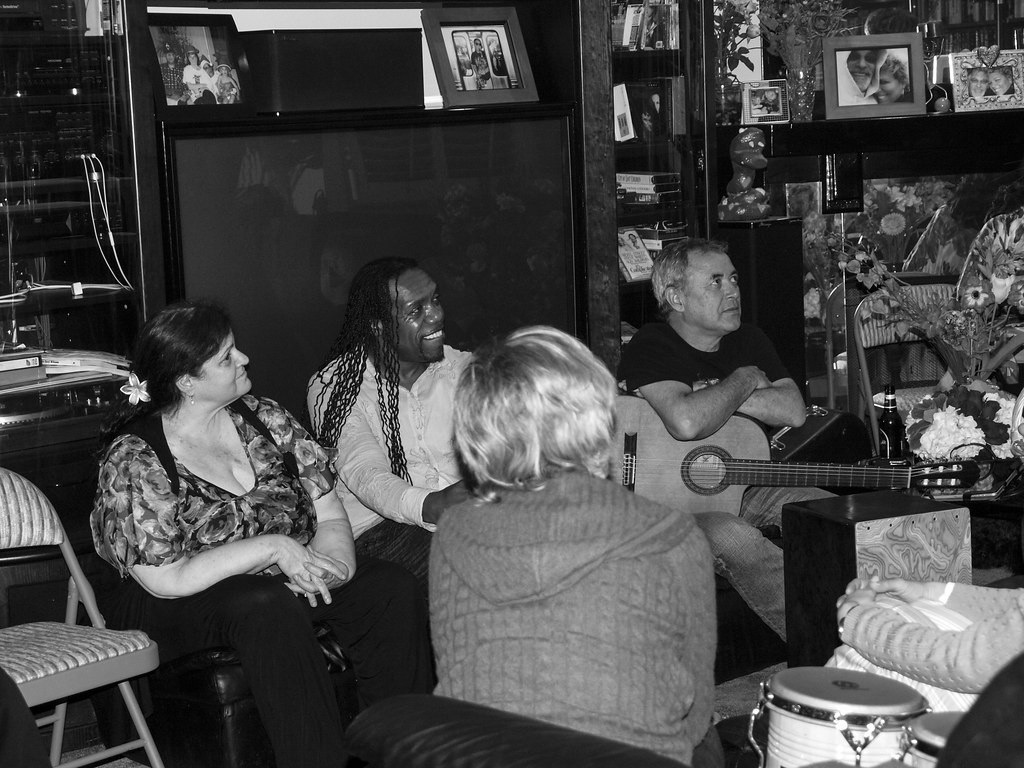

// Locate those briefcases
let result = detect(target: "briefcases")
[767,404,872,461]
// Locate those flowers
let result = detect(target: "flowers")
[120,373,152,405]
[713,1,771,72]
[758,1,864,69]
[818,215,1024,462]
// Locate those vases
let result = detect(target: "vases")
[713,58,743,125]
[786,67,816,123]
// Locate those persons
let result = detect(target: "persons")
[752,90,781,116]
[157,43,240,106]
[93,295,428,768]
[966,66,1013,96]
[625,235,844,643]
[470,37,499,89]
[788,184,816,218]
[835,574,1024,694]
[304,249,483,690]
[836,49,911,106]
[428,321,719,768]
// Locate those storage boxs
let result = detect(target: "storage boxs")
[240,28,423,113]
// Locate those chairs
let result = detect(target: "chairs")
[0,465,165,768]
[853,283,958,456]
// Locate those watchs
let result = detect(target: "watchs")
[837,603,860,640]
[703,376,720,389]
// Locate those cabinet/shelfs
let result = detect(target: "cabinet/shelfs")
[0,0,178,757]
[607,0,1024,342]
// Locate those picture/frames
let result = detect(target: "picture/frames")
[146,12,259,121]
[822,32,927,120]
[420,7,540,108]
[741,79,790,126]
[949,50,1024,112]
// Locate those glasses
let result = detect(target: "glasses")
[847,51,878,64]
[474,43,481,46]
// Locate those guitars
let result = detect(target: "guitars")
[609,395,991,517]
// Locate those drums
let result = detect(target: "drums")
[748,665,930,768]
[901,711,966,768]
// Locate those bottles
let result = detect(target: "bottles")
[879,383,905,460]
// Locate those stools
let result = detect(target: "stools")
[100,624,359,768]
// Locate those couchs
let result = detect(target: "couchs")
[342,567,1024,768]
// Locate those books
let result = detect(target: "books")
[763,152,837,213]
[611,0,680,51]
[611,73,686,142]
[616,225,657,282]
[0,345,133,394]
